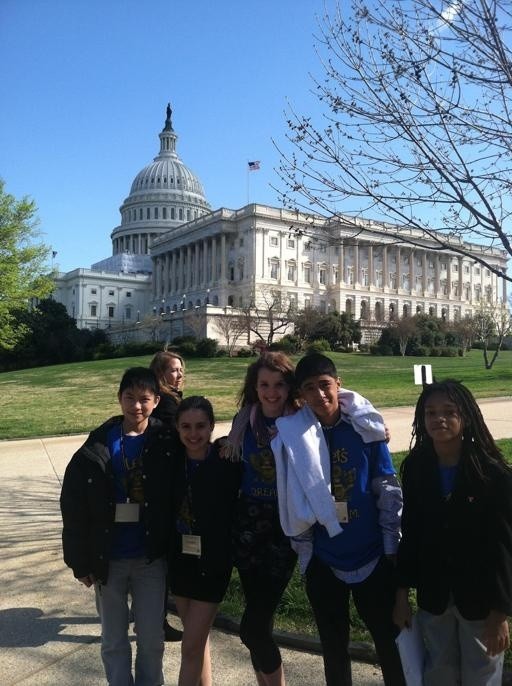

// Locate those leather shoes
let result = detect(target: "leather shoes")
[162,619,184,642]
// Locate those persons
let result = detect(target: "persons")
[128,352,185,640]
[213,352,303,686]
[389,379,512,686]
[60,366,174,686]
[165,396,243,686]
[270,351,403,686]
